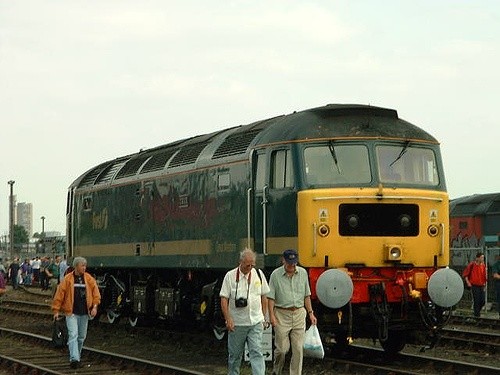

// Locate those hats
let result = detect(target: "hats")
[282,249,298,264]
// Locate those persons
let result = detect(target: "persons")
[462,252,487,317]
[52,256,102,369]
[266,249,317,375]
[220,248,271,375]
[0,255,70,296]
[492,258,500,319]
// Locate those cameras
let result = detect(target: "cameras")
[234,299,247,308]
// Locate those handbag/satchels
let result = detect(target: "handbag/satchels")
[303,324,325,359]
[50,320,68,349]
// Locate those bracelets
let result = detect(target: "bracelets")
[308,310,314,313]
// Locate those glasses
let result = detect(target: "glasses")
[245,264,255,267]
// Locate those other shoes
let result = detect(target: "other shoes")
[70,360,82,370]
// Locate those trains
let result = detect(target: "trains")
[449,194,500,301]
[65,103,465,355]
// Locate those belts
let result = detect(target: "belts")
[274,305,304,311]
